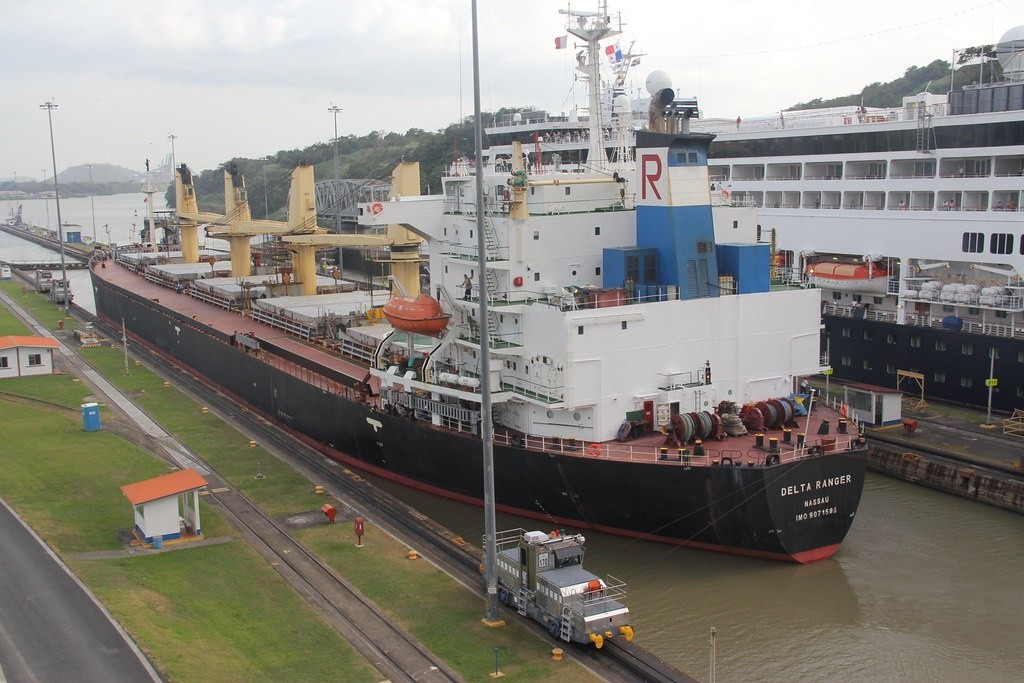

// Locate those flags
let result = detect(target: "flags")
[606,43,619,55]
[554,35,567,49]
[609,50,622,63]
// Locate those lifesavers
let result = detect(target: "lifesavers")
[372,203,383,213]
[721,190,729,199]
[588,443,603,456]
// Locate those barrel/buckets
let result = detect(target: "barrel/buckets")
[633,284,676,302]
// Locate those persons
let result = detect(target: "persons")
[460,274,472,301]
[801,379,808,394]
[736,116,741,132]
[780,113,785,128]
[856,106,867,124]
[541,128,609,142]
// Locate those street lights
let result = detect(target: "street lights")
[87,165,97,244]
[41,169,50,239]
[40,102,72,320]
[167,134,176,181]
[328,105,345,280]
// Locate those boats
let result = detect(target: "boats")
[632,40,1024,416]
[91,1,869,566]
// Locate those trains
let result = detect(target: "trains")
[481,527,633,649]
[35,270,74,303]
